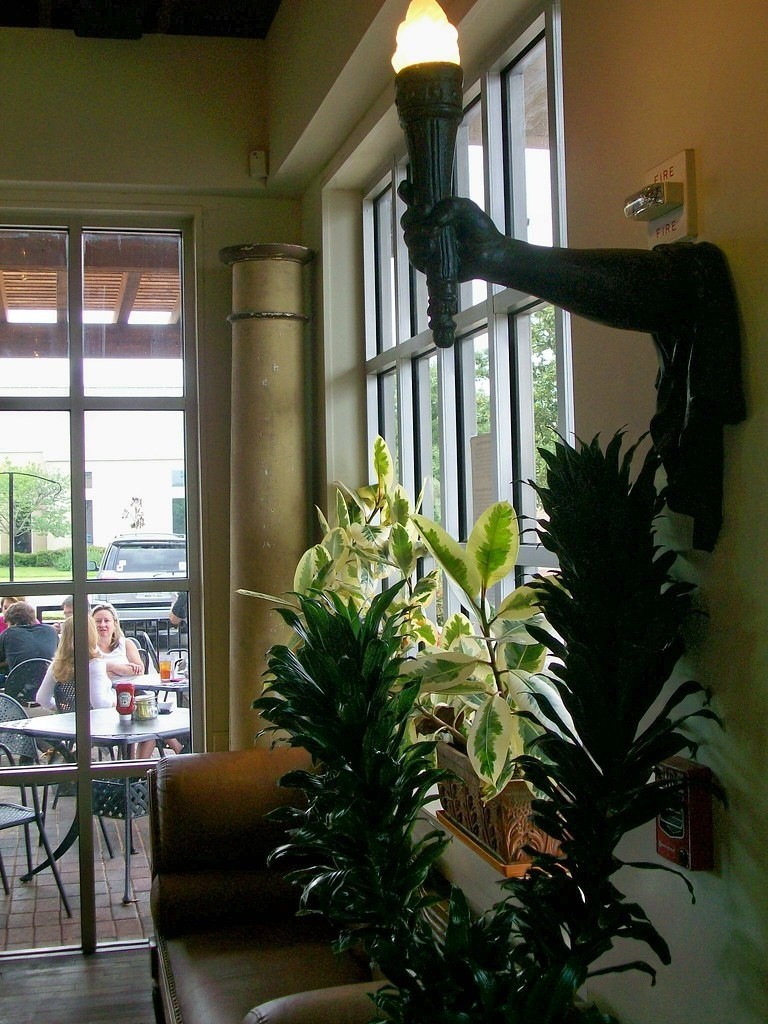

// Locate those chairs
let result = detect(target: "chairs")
[0,637,192,918]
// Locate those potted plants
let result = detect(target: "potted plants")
[389,499,563,878]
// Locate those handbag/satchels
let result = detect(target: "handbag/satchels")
[40,747,64,764]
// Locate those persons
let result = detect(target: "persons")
[168,591,189,625]
[398,179,680,335]
[52,596,74,633]
[0,596,40,634]
[34,614,135,758]
[0,601,60,765]
[90,603,184,759]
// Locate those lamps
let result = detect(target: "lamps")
[391,0,751,553]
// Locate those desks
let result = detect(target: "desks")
[0,707,191,907]
[111,674,189,709]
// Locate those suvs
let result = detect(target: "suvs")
[87,533,188,649]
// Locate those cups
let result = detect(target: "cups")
[159,660,171,682]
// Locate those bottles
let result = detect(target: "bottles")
[114,682,134,722]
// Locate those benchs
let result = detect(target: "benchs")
[146,743,461,1023]
